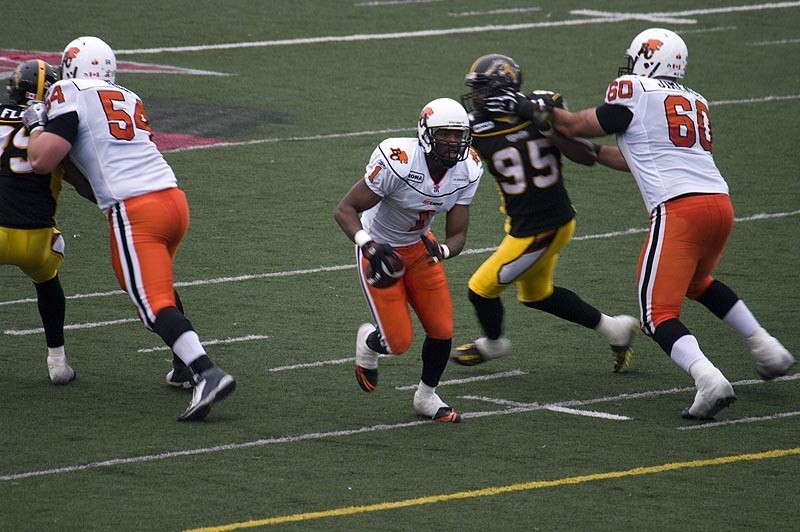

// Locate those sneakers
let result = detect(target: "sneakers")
[681,376,736,422]
[178,369,236,423]
[413,393,461,423]
[450,338,510,366]
[50,365,77,386]
[610,315,640,374]
[756,351,796,382]
[355,322,379,392]
[166,369,205,392]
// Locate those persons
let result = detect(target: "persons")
[0,59,98,385]
[483,28,796,419]
[24,36,236,422]
[461,54,640,372]
[332,98,485,424]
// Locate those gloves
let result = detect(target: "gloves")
[421,231,450,264]
[23,103,47,135]
[528,90,568,111]
[562,136,600,166]
[362,242,405,288]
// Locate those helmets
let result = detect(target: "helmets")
[619,28,688,79]
[7,60,59,104]
[418,98,473,163]
[61,36,116,82]
[461,53,523,113]
